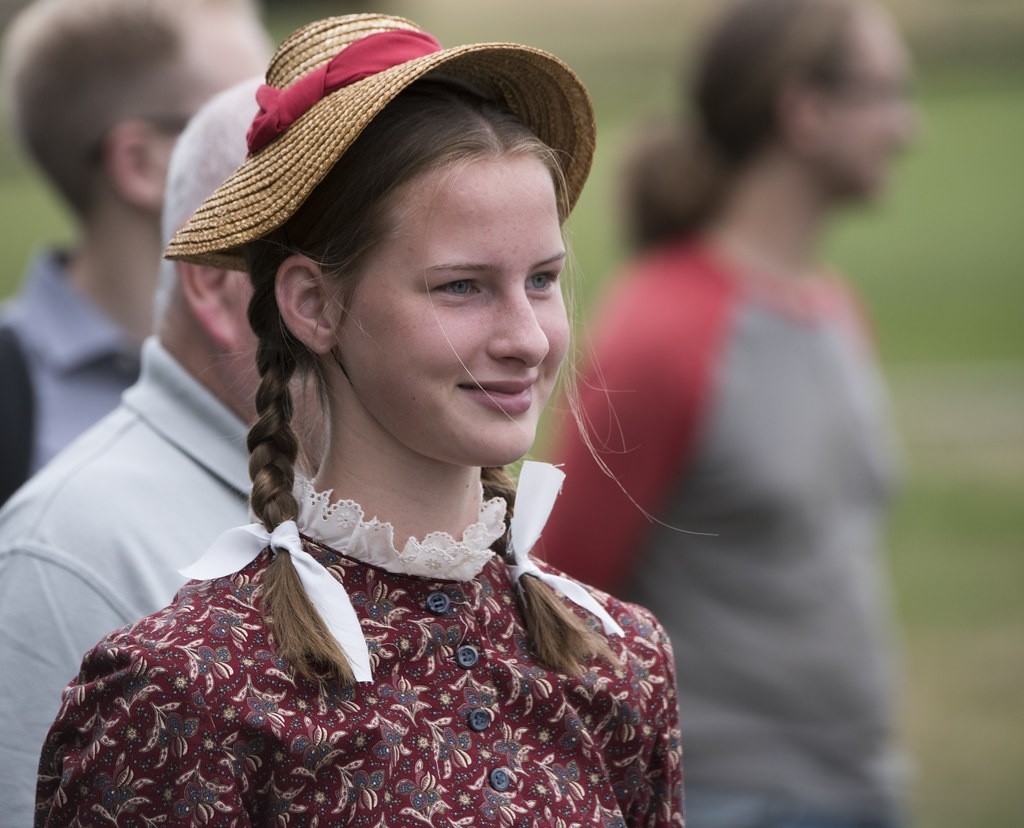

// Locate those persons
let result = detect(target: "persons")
[34,12,693,828]
[0,75,290,828]
[535,1,922,828]
[0,0,278,500]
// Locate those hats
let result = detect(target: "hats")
[163,12,597,273]
